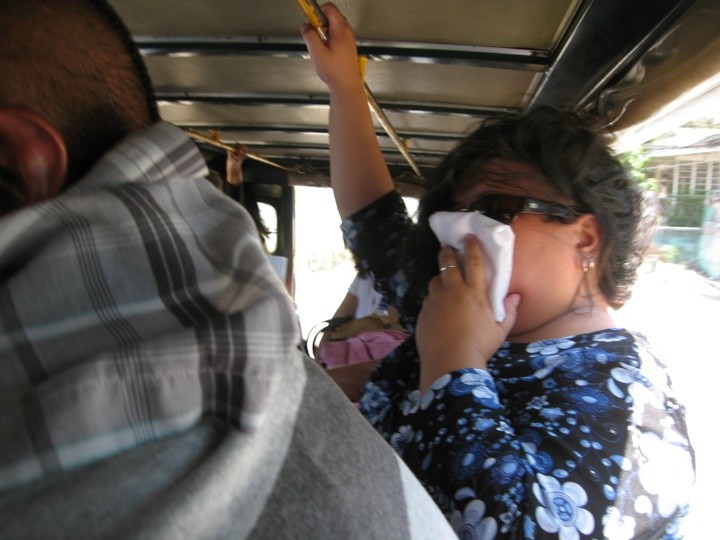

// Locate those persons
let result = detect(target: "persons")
[300,3,695,540]
[2,0,460,540]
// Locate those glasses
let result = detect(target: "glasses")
[447,192,582,231]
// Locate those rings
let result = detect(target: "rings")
[441,265,458,271]
